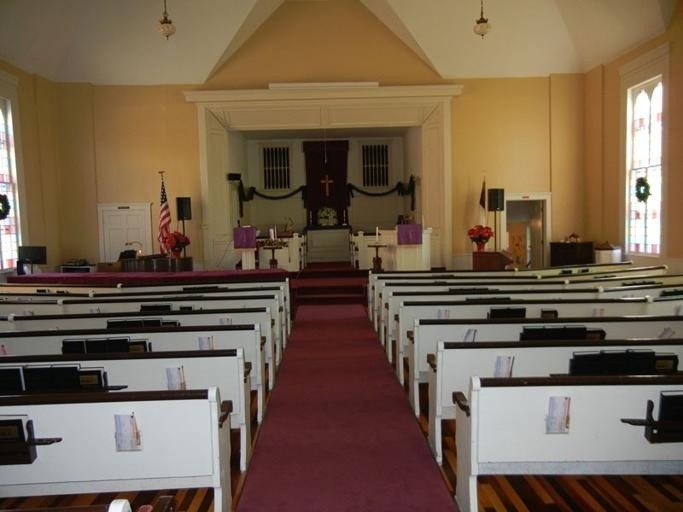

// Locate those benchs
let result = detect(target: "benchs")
[0,277,291,424]
[367,267,683,386]
[1,347,252,512]
[407,315,682,512]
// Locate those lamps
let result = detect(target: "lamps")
[158,1,176,41]
[473,0,492,39]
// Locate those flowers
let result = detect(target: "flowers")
[467,224,494,240]
[162,231,191,251]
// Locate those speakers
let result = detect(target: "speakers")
[175,196,193,222]
[19,245,47,264]
[488,188,504,211]
[227,172,241,183]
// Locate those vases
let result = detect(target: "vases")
[171,248,182,256]
[477,241,485,253]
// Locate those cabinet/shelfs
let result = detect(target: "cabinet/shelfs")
[473,252,505,271]
[550,242,594,267]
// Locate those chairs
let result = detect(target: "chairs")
[119,249,137,260]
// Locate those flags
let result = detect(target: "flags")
[157,174,171,254]
[478,180,486,209]
